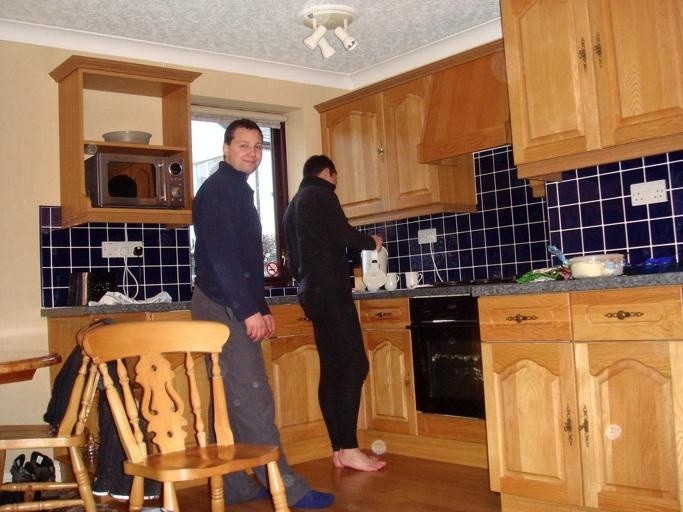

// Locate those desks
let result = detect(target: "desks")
[1,354,64,386]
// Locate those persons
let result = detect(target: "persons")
[190,119,335,509]
[280,154,387,471]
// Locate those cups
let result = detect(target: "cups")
[68,271,94,306]
[354,276,366,291]
[385,271,400,291]
[405,271,425,289]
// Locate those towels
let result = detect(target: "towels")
[90,286,174,307]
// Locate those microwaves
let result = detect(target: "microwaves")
[83,152,184,212]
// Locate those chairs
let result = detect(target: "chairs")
[82,320,296,512]
[0,316,115,512]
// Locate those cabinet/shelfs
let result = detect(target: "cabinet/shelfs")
[361,299,418,440]
[60,69,193,233]
[478,285,681,512]
[499,0,682,197]
[267,304,362,432]
[319,74,479,229]
[51,308,209,455]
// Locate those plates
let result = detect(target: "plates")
[622,264,676,274]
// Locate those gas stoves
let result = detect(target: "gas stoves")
[420,276,514,287]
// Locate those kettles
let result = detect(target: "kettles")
[360,246,388,289]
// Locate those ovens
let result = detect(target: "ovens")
[410,295,485,421]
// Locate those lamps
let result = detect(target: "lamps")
[299,5,359,61]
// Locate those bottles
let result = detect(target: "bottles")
[85,433,95,467]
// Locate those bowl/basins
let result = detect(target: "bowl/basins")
[101,130,153,143]
[568,253,624,280]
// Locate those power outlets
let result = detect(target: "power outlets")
[629,178,668,207]
[101,240,145,258]
[417,227,437,244]
